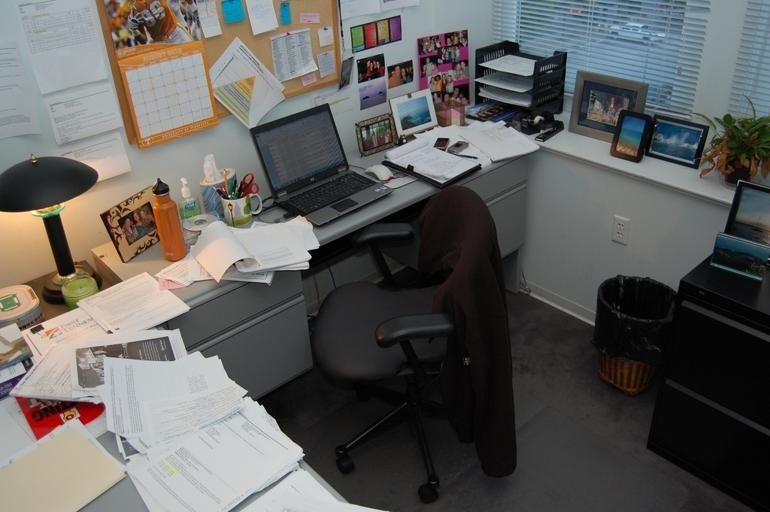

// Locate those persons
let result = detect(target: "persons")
[358,31,468,105]
[589,95,630,126]
[122,209,154,243]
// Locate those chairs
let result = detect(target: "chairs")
[305,175,502,507]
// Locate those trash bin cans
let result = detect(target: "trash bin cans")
[596,275,679,398]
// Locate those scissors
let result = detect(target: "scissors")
[240,173,259,198]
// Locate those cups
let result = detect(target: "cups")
[222,194,264,227]
[199,168,236,218]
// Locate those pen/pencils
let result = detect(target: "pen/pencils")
[213,169,239,199]
[455,154,477,159]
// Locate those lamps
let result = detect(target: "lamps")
[0,152,105,306]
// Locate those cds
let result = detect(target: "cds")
[183,214,218,231]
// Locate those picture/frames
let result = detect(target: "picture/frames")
[568,68,650,144]
[643,112,710,169]
[608,107,654,164]
[97,184,159,264]
[355,112,399,157]
[388,87,439,140]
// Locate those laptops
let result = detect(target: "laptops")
[250,103,394,227]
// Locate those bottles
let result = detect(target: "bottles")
[152,178,188,260]
[61,272,99,308]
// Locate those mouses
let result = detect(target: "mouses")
[366,164,394,181]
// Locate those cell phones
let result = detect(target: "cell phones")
[448,141,468,154]
[434,138,449,150]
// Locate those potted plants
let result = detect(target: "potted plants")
[692,94,769,187]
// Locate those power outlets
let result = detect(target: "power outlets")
[611,213,631,247]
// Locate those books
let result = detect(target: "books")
[1,301,147,445]
[382,138,482,187]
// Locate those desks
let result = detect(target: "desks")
[0,258,350,511]
[86,120,540,407]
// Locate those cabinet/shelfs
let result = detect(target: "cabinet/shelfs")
[644,252,770,512]
[472,39,569,136]
